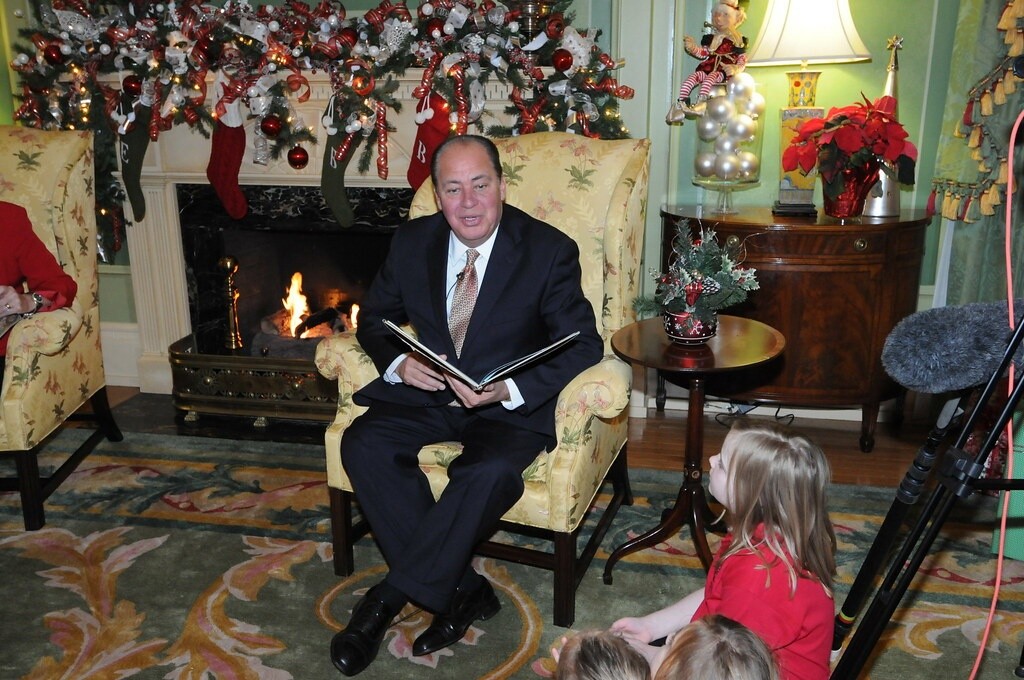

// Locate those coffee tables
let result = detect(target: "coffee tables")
[604,311,787,586]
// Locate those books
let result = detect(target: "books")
[382,319,581,394]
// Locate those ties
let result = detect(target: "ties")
[448,248,478,358]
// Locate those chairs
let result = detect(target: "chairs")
[315,132,652,630]
[0,122,122,532]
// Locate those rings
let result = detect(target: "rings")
[5,303,11,309]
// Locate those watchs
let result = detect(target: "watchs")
[29,292,44,314]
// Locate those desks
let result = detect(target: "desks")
[658,204,930,450]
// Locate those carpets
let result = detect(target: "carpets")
[0,430,1023,679]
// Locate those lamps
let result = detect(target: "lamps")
[743,0,873,214]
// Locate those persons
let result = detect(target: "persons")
[650,613,781,680]
[330,135,604,675]
[0,199,78,399]
[552,628,652,680]
[610,418,838,680]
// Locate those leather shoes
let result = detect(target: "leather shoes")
[330,585,400,676]
[412,575,501,656]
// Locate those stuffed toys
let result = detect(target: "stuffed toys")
[664,0,750,126]
